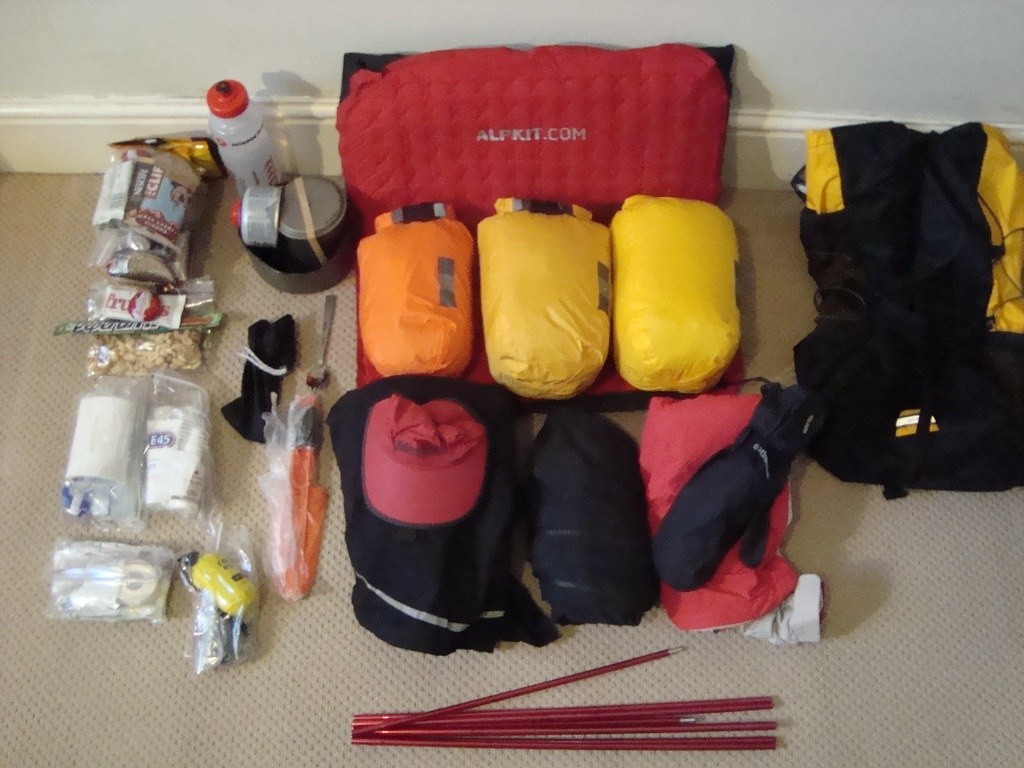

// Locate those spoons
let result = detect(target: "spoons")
[305,295,340,389]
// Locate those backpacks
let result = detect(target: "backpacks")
[785,120,1024,496]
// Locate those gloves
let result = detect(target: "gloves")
[651,381,829,591]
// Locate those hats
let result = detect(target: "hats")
[361,395,491,530]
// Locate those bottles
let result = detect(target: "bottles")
[207,80,283,196]
